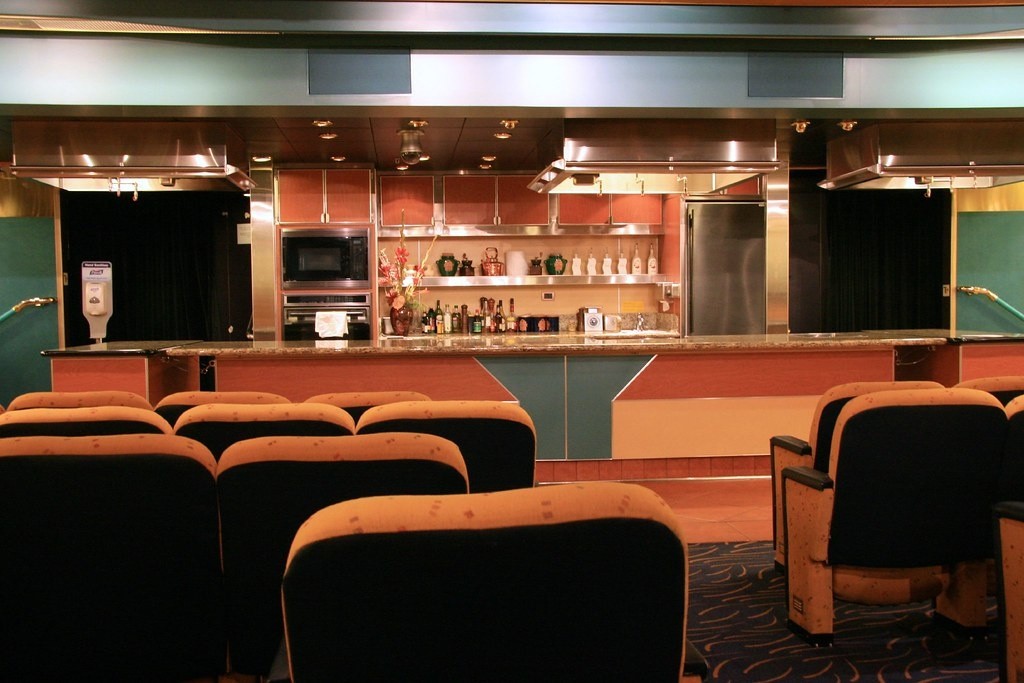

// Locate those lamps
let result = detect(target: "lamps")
[839,121,857,131]
[791,120,810,132]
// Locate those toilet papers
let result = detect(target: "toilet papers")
[504,251,529,276]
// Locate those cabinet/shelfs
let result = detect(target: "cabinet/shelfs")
[273,164,663,342]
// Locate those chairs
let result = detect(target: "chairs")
[771,376,1024,683]
[1,391,710,683]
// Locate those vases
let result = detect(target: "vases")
[390,305,413,338]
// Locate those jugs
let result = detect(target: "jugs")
[480,247,505,276]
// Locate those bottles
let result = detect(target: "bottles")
[421,309,428,334]
[444,304,452,334]
[505,298,519,334]
[617,254,627,274]
[428,308,436,334]
[436,253,460,276]
[462,305,469,333]
[572,255,581,275]
[473,310,482,333]
[602,253,612,275]
[495,300,507,333]
[452,305,462,333]
[586,253,596,275]
[544,256,568,275]
[632,243,641,274]
[480,297,495,333]
[436,300,444,333]
[530,259,542,275]
[647,243,658,274]
[459,260,474,276]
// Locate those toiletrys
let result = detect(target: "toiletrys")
[572,242,658,275]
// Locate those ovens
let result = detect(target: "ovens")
[283,237,368,282]
[284,293,370,339]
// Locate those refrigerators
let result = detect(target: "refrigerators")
[686,195,767,335]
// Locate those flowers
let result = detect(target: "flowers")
[377,208,439,310]
